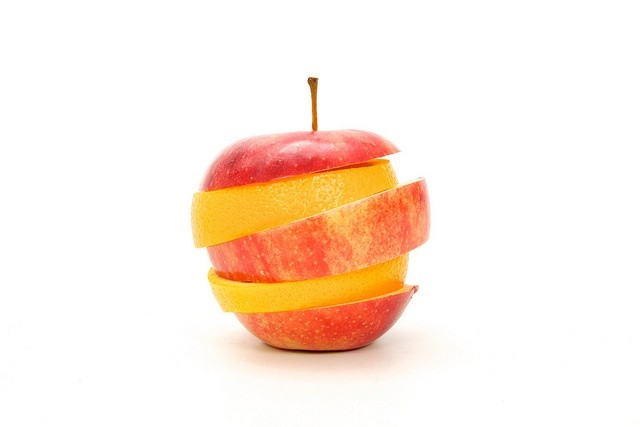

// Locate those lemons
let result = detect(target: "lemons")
[191,160,398,248]
[207,254,410,312]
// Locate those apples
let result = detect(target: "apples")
[201,78,401,190]
[230,286,416,350]
[207,177,429,283]
